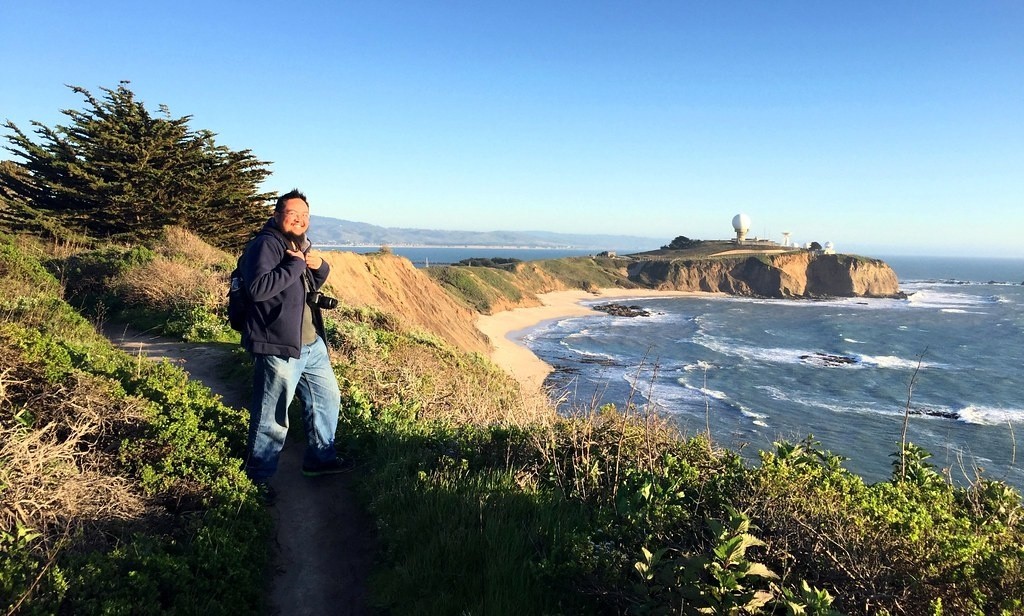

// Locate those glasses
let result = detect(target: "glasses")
[283,212,311,219]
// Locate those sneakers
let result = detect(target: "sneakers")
[303,457,355,476]
[256,481,279,506]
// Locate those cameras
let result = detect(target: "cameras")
[306,292,338,309]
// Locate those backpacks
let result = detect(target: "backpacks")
[224,229,298,332]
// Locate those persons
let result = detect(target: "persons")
[236,188,357,509]
